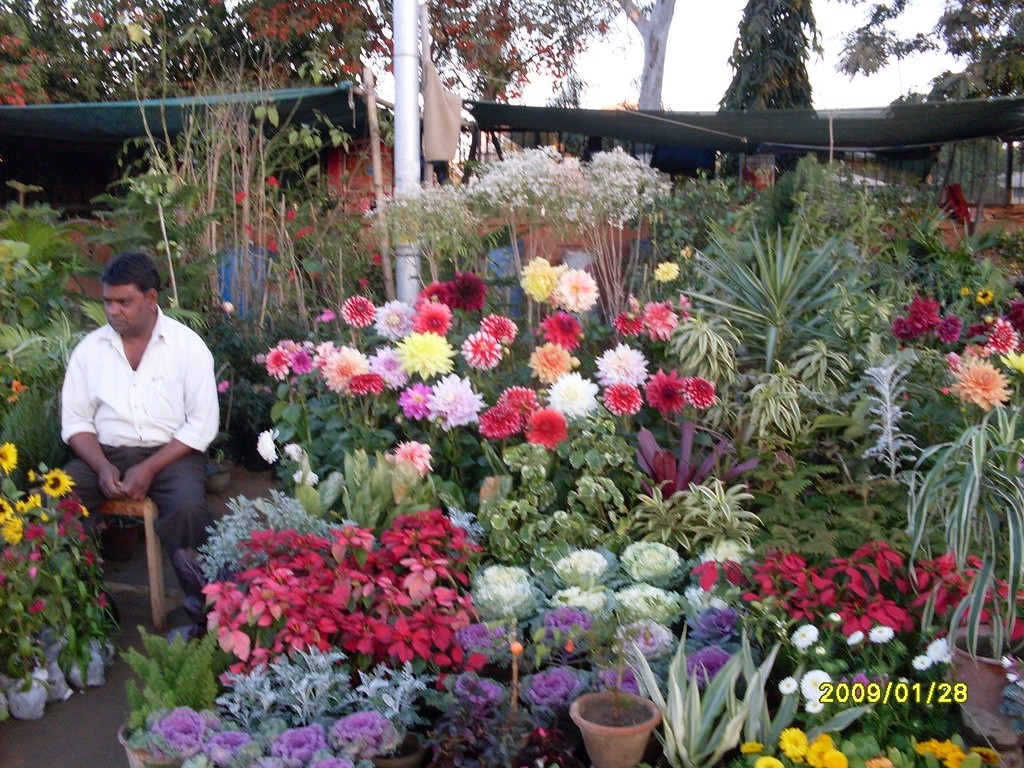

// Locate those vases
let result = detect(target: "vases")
[372,733,427,768]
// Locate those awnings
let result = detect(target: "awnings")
[454,92,1024,209]
[1,81,408,171]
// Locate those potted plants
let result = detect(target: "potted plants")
[117,627,221,768]
[567,611,663,768]
[905,401,1024,749]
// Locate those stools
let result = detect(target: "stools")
[101,498,187,630]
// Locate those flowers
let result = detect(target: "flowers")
[0,145,1023,767]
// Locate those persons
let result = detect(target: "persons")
[57,249,220,687]
